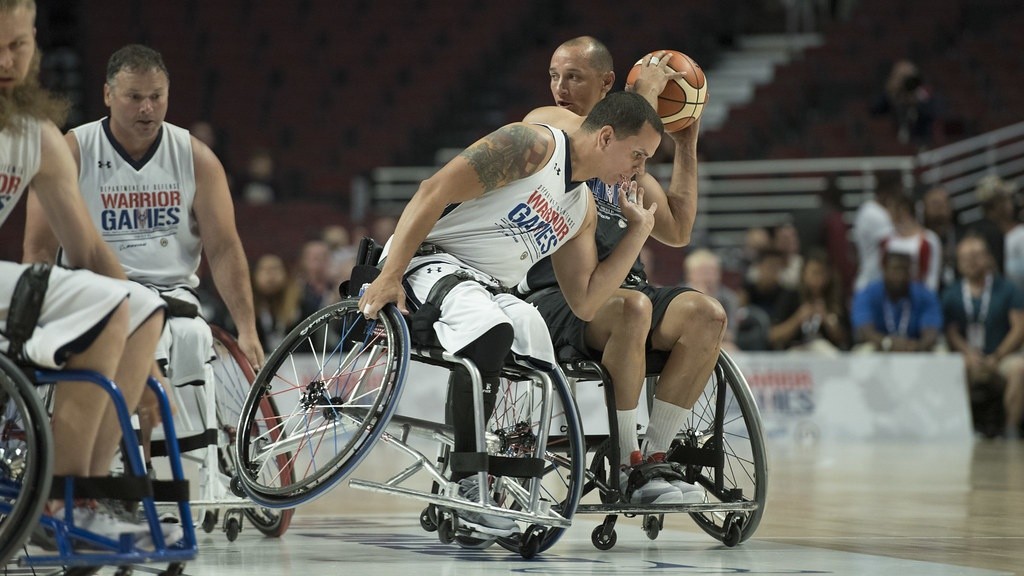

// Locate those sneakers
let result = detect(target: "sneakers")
[434,474,519,537]
[607,450,684,504]
[32,501,184,553]
[646,452,704,502]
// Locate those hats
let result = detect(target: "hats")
[974,175,1017,201]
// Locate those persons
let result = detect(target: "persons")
[22,42,265,479]
[179,122,1024,443]
[0,0,184,552]
[358,36,727,537]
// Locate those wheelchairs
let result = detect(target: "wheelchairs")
[231,237,768,562]
[0,261,298,576]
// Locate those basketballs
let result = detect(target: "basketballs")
[623,47,710,135]
